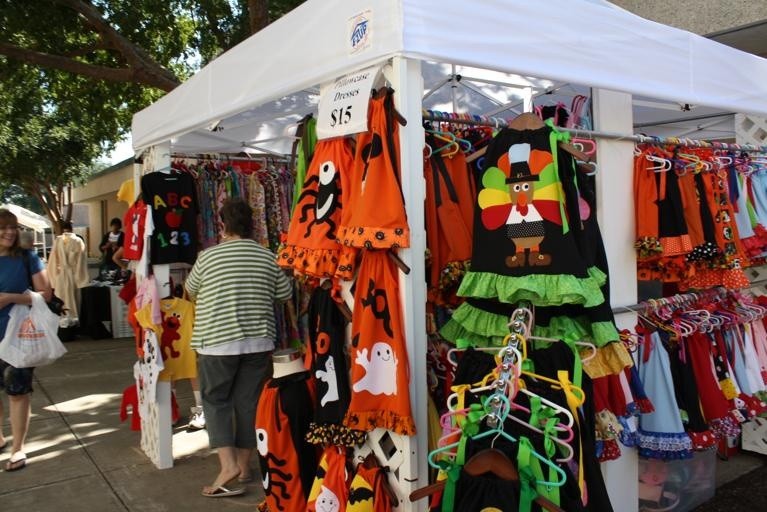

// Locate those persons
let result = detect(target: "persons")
[15,231,36,263]
[97,217,125,278]
[41,222,91,328]
[0,206,52,471]
[181,199,294,500]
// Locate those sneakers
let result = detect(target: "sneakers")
[187,405,206,430]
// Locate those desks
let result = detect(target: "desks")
[81,284,131,338]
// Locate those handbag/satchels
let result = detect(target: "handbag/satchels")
[20,248,68,317]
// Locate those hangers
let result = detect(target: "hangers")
[409,307,596,512]
[640,286,767,342]
[142,152,297,181]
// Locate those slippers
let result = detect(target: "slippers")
[5,450,28,472]
[202,483,246,498]
